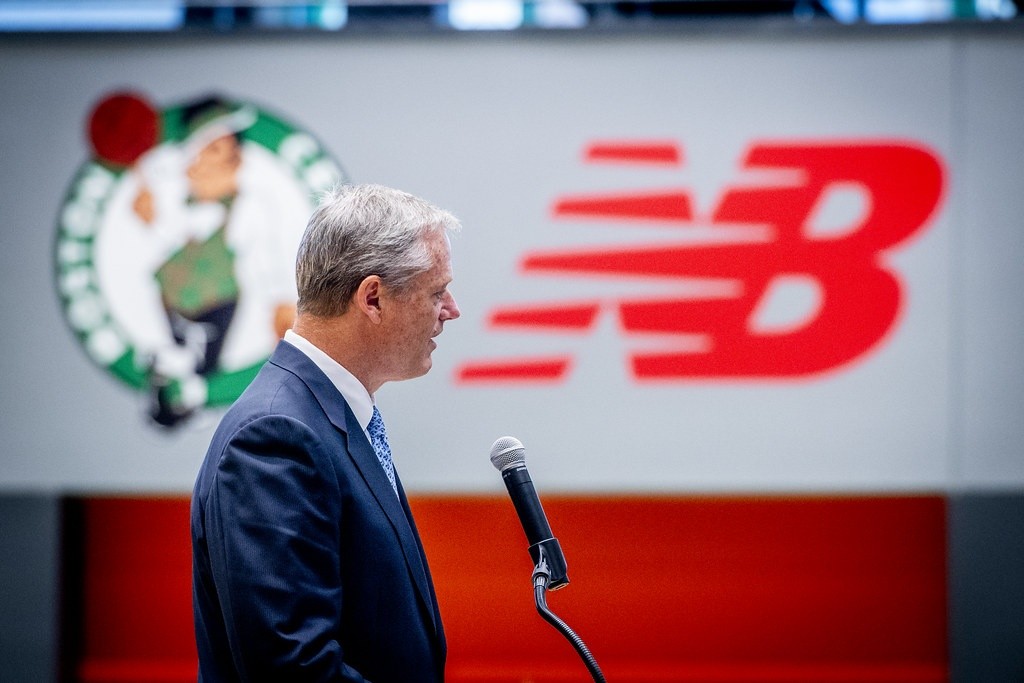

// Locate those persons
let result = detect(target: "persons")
[191,185,462,683]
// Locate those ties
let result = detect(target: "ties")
[366,405,400,503]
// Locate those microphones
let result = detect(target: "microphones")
[490,436,570,591]
